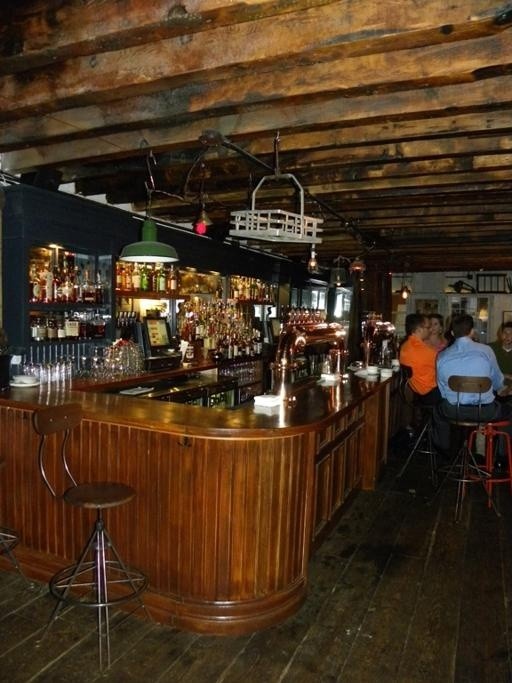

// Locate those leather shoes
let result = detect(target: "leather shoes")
[493,459,508,474]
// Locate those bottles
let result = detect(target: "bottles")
[174,352,335,409]
[27,246,275,359]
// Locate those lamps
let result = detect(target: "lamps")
[194,164,214,228]
[120,172,182,264]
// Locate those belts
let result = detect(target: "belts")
[448,400,491,409]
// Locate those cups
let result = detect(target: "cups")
[78,345,144,379]
[366,359,400,379]
[8,353,75,389]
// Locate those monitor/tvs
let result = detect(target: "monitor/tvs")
[143,316,172,349]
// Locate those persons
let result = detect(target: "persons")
[423,310,448,351]
[487,321,511,376]
[399,313,447,433]
[431,314,511,456]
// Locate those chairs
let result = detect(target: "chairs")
[396,362,512,526]
[26,403,160,678]
